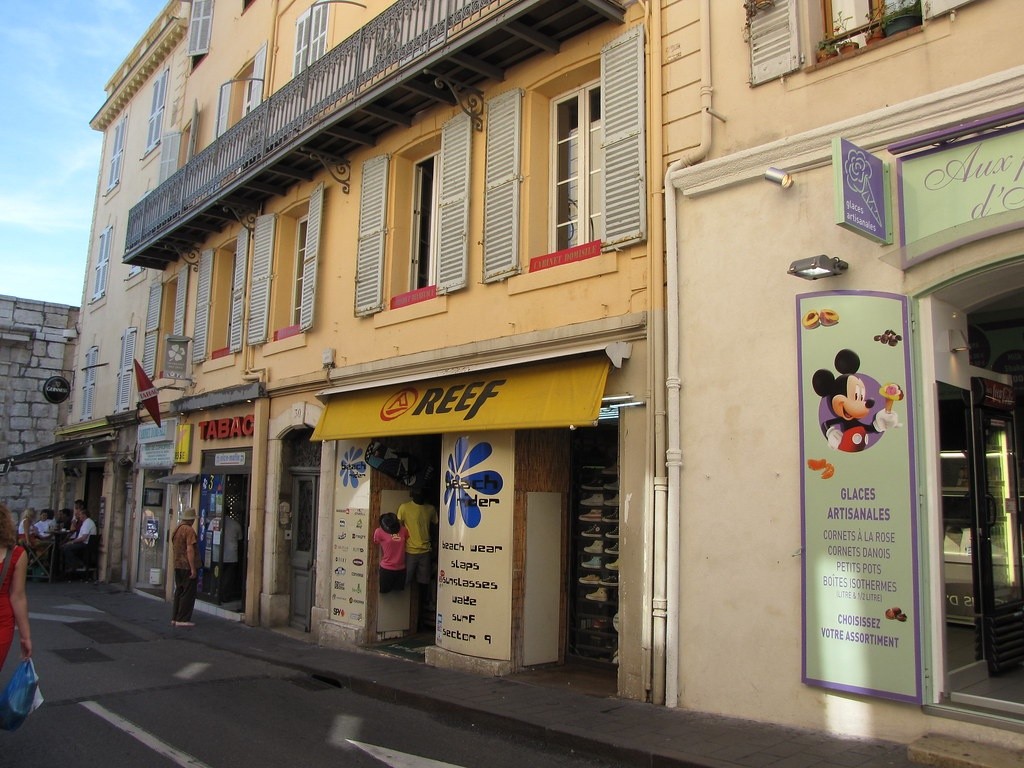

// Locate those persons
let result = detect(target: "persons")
[172,508,201,626]
[373,513,409,593]
[208,505,243,603]
[0,503,33,671]
[397,481,438,584]
[18,499,96,568]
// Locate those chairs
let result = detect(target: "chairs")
[61,533,99,583]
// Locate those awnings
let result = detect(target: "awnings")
[0,434,113,477]
[309,356,610,441]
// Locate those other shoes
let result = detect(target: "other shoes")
[172,619,194,627]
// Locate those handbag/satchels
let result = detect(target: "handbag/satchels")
[0,656,44,732]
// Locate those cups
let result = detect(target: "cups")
[49,525,53,531]
[55,524,59,530]
[60,523,64,529]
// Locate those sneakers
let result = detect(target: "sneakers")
[581,448,621,601]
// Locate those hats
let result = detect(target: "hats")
[380,511,401,534]
[410,484,429,505]
[179,508,200,520]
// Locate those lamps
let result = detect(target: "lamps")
[766,167,792,188]
[786,254,849,280]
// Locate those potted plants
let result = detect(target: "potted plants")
[816,37,837,62]
[882,3,922,37]
[863,1,894,45]
[833,11,859,55]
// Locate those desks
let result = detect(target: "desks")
[42,529,77,584]
